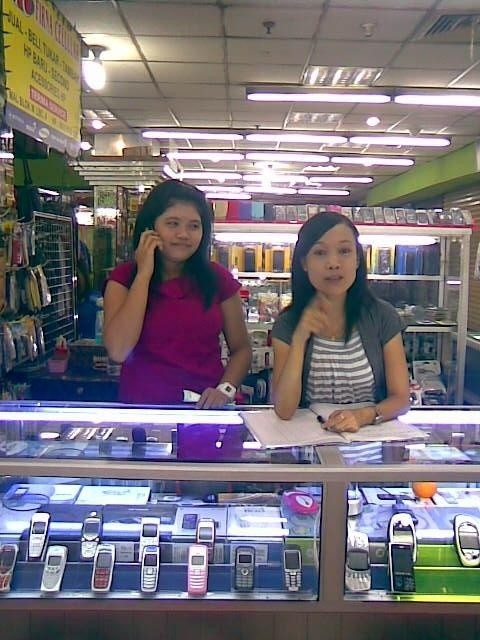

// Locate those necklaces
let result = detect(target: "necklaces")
[326,312,343,342]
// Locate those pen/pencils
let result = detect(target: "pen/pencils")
[317,416,328,431]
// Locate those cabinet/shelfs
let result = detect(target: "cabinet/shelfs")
[209,222,471,406]
[0,404,480,640]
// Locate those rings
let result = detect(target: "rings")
[339,414,345,421]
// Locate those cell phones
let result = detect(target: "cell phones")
[182,514,197,529]
[139,517,160,563]
[196,518,215,564]
[9,487,29,500]
[283,547,303,593]
[389,513,417,563]
[40,546,68,593]
[377,494,411,500]
[80,517,102,561]
[187,545,208,596]
[234,545,256,590]
[392,504,418,524]
[439,492,458,504]
[91,543,116,593]
[389,543,416,593]
[142,221,158,252]
[26,512,51,562]
[455,515,480,567]
[0,544,19,593]
[140,545,161,593]
[208,200,475,275]
[345,532,371,591]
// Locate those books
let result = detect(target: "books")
[238,401,430,449]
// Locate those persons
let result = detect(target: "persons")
[266,210,412,466]
[102,179,255,459]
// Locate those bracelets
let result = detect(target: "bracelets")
[216,381,236,401]
[369,405,383,424]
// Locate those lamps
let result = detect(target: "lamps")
[391,87,480,107]
[245,130,349,144]
[309,175,374,184]
[243,185,296,196]
[297,188,350,197]
[246,150,330,163]
[167,150,244,162]
[197,186,251,200]
[142,127,247,140]
[331,153,414,167]
[241,174,308,184]
[245,86,396,104]
[179,169,242,181]
[347,132,454,147]
[81,43,108,91]
